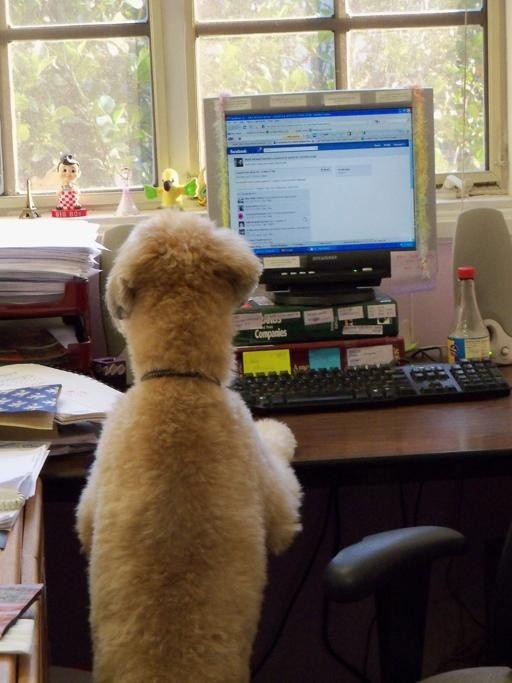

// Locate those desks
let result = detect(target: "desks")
[40,358,512,531]
[1,422,51,683]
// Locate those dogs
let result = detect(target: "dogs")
[75,209,304,683]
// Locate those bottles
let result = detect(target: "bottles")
[446,266,492,363]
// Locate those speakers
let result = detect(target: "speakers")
[100,223,139,358]
[451,206,512,366]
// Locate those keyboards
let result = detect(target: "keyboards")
[224,356,512,418]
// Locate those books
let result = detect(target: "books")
[0,384,63,431]
[0,363,128,423]
[1,279,70,295]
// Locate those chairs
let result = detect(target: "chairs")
[315,522,512,682]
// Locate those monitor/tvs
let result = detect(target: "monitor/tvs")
[200,87,438,309]
